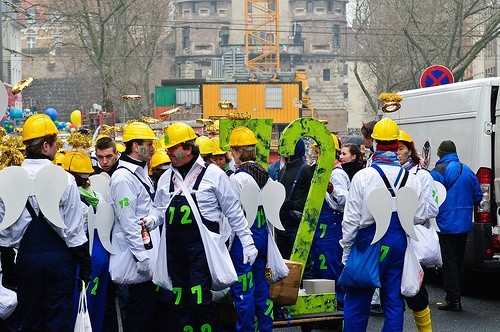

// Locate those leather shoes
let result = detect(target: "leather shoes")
[438,299,462,312]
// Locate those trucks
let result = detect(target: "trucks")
[372,78,499,281]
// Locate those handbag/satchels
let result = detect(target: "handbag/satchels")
[266,230,289,284]
[74,280,93,332]
[0,274,18,319]
[336,239,381,289]
[199,224,238,292]
[109,223,160,285]
[401,225,443,297]
[152,226,172,291]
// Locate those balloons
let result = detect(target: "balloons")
[44,108,57,121]
[11,109,22,119]
[69,110,81,125]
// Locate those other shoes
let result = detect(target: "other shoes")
[370,304,384,315]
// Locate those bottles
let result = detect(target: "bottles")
[139,218,153,250]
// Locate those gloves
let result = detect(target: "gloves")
[242,245,259,265]
[75,241,92,291]
[138,216,154,232]
[0,247,16,290]
[136,259,150,278]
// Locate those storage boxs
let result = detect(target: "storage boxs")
[303,278,335,294]
[267,259,302,305]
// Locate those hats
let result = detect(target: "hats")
[437,140,456,157]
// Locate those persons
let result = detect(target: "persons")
[429,140,483,312]
[0,114,439,332]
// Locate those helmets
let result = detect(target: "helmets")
[55,150,94,173]
[21,114,60,142]
[228,127,258,146]
[309,133,341,152]
[95,134,125,152]
[195,136,227,156]
[122,121,198,175]
[370,118,413,142]
